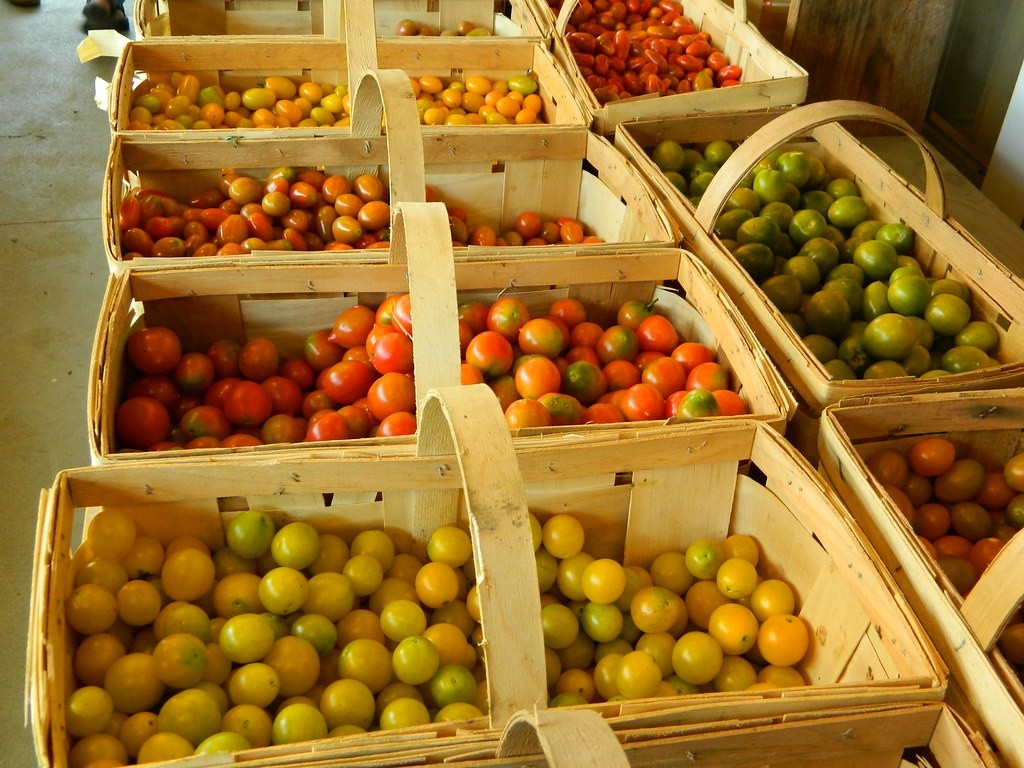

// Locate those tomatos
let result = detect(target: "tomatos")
[62,0,1024,768]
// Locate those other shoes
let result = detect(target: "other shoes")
[82,0,131,35]
